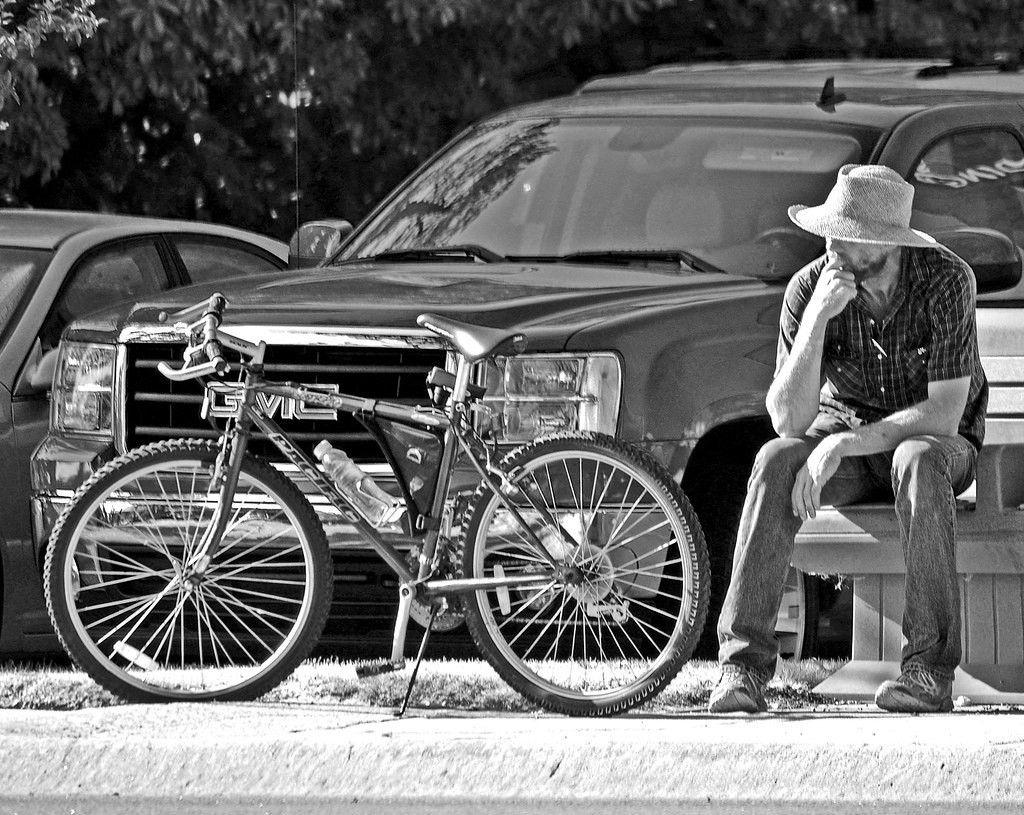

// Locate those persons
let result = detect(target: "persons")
[708,162,990,712]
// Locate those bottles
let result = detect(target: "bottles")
[312,438,395,522]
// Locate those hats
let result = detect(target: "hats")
[787,163,940,249]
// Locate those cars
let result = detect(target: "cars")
[0,209,292,667]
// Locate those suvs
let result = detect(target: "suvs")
[24,57,1024,662]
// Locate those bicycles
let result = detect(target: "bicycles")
[43,287,714,721]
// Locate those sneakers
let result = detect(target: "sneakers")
[874,667,954,714]
[707,661,770,714]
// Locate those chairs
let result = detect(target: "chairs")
[640,180,726,268]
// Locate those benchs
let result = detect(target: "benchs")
[786,309,1023,705]
